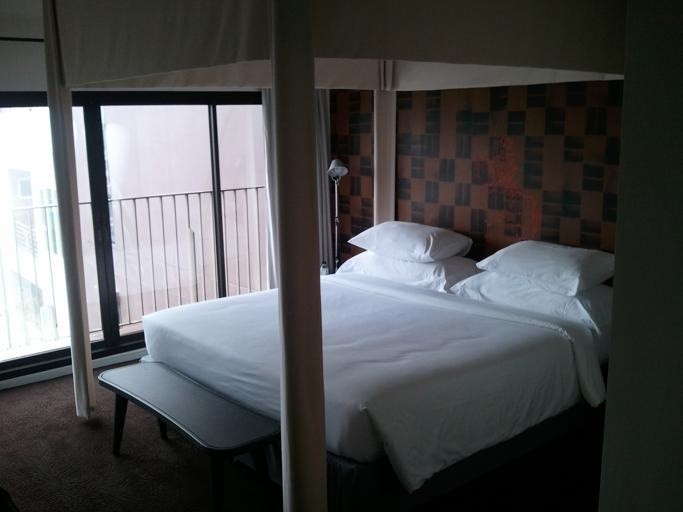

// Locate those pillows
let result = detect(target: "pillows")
[336,221,615,331]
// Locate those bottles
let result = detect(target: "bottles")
[319,260,329,275]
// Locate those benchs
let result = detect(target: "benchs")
[97,361,279,512]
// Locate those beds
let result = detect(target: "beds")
[142,275,610,511]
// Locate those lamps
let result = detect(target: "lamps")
[326,159,349,273]
